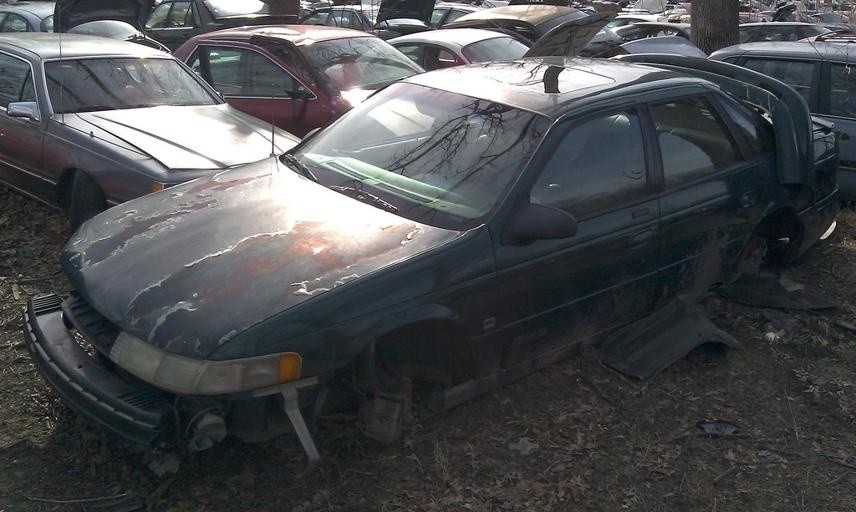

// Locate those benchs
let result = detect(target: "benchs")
[593,111,736,177]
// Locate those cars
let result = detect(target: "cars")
[0,31,305,237]
[18,50,824,454]
[0,2,856,141]
[353,27,527,84]
[170,23,431,141]
[704,29,856,204]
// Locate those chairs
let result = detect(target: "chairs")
[658,131,712,177]
